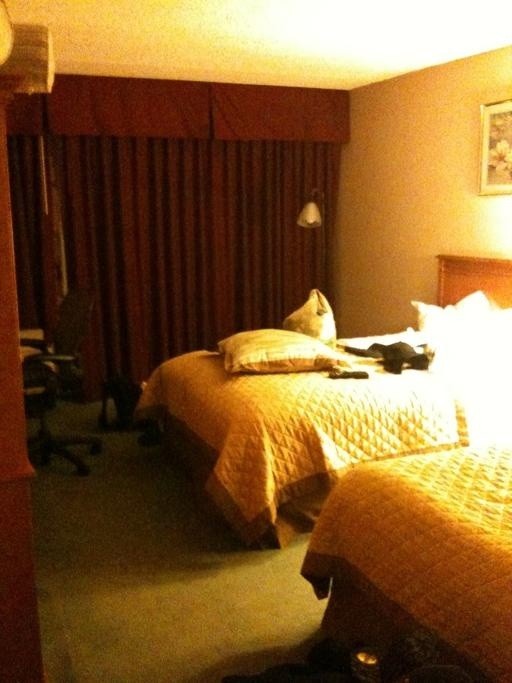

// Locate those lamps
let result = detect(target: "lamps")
[295,186,324,299]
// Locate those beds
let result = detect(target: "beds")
[299,441,511,680]
[133,254,511,550]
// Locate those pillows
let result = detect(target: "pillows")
[406,290,500,329]
[216,329,349,376]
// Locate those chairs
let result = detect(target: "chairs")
[21,276,103,476]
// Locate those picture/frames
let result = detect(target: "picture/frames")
[478,99,511,195]
